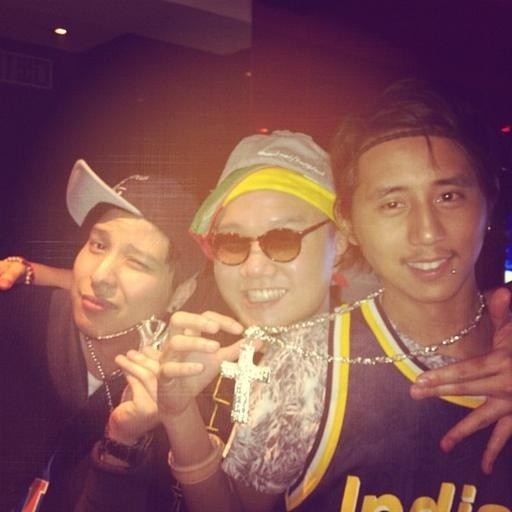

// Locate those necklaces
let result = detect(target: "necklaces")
[83,313,165,417]
[219,278,486,425]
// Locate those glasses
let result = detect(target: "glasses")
[206,208,331,265]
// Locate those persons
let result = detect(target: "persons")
[154,74,511,512]
[0,157,213,512]
[147,125,352,512]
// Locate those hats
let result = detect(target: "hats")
[187,129,358,261]
[66,159,208,272]
[330,77,496,186]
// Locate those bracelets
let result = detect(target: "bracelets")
[167,431,226,487]
[4,255,35,286]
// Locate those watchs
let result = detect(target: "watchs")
[99,431,166,472]
